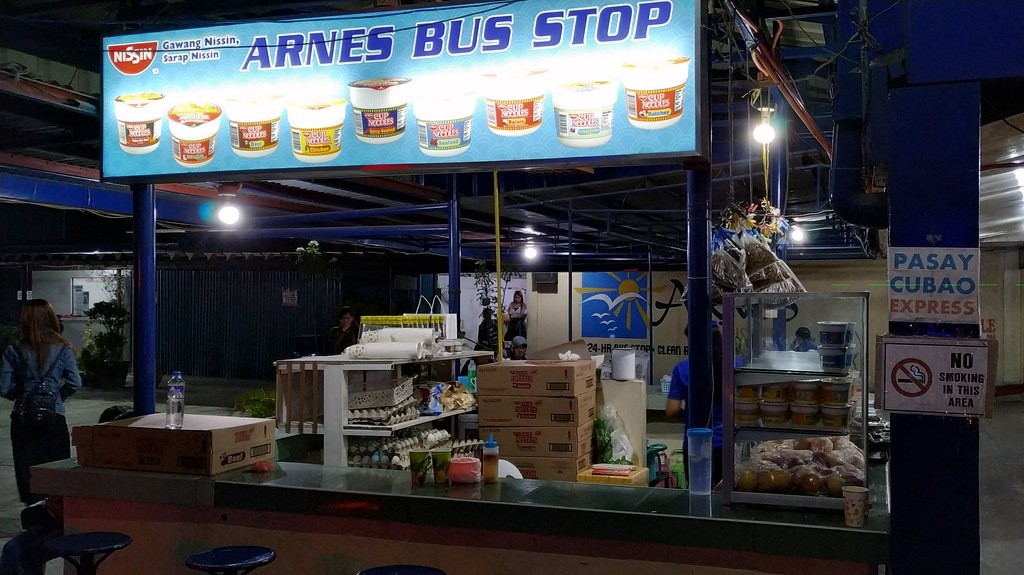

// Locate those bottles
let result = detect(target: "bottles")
[482,433,499,483]
[357,315,463,356]
[687,428,713,496]
[165,371,186,429]
[689,494,712,519]
[468,359,477,391]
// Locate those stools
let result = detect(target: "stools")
[185,545,278,575]
[44,532,134,575]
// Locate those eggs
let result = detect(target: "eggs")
[349,426,448,464]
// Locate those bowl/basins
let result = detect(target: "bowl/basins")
[736,319,860,427]
[449,458,480,482]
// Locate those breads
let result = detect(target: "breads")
[741,435,864,496]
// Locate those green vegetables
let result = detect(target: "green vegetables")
[593,412,633,464]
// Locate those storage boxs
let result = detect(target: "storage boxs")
[73,415,277,476]
[477,360,593,483]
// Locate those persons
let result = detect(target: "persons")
[502,336,527,361]
[0,300,82,507]
[666,321,746,491]
[790,326,818,352]
[326,305,360,356]
[461,308,499,376]
[507,290,527,324]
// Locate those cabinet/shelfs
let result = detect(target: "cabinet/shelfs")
[724,292,869,513]
[276,350,497,474]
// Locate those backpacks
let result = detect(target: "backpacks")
[12,343,67,426]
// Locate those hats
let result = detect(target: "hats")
[512,336,527,349]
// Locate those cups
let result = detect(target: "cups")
[842,486,869,527]
[409,448,429,485]
[431,448,452,483]
[113,49,689,169]
[347,326,435,359]
